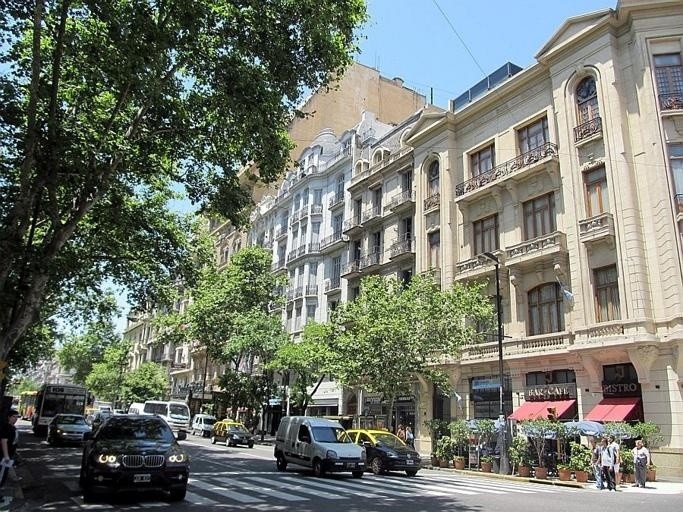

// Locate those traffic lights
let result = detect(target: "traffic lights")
[546,406,557,424]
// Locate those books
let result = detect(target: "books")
[0,458,14,468]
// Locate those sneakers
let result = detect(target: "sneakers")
[607,482,645,491]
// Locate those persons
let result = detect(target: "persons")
[0,409,23,496]
[587,437,604,488]
[607,434,621,486]
[632,440,650,488]
[396,424,404,440]
[405,427,413,443]
[597,438,615,492]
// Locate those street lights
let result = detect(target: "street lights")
[194,343,208,413]
[483,251,507,425]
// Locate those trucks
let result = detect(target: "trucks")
[273,415,366,479]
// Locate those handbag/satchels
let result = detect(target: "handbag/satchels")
[636,457,647,469]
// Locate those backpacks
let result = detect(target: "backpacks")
[607,446,617,465]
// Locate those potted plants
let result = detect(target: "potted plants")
[416,413,665,489]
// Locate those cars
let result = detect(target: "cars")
[337,428,420,476]
[9,383,257,502]
